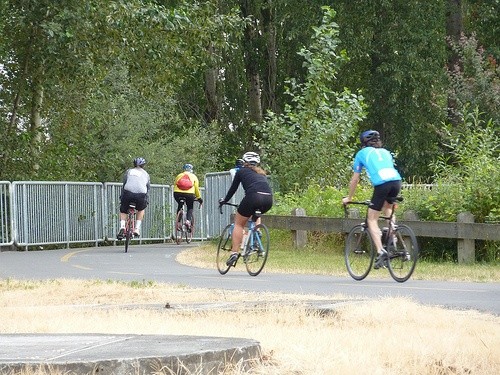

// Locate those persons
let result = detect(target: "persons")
[173,164,203,233]
[219,152,274,267]
[117,157,151,240]
[343,131,403,269]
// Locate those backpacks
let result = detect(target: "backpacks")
[177,174,192,190]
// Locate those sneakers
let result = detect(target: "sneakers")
[391,237,398,245]
[374,249,388,269]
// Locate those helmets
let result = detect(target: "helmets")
[242,152,260,164]
[236,159,244,164]
[183,164,193,169]
[134,157,145,166]
[360,130,379,142]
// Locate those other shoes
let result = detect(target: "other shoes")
[185,222,191,233]
[117,228,125,240]
[177,225,182,231]
[256,231,262,240]
[226,253,238,266]
[133,230,140,237]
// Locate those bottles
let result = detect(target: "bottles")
[240,232,248,250]
[381,227,389,244]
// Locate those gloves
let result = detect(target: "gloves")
[198,198,203,204]
[219,201,224,207]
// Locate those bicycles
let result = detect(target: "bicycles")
[174,196,203,244]
[117,196,149,253]
[216,198,269,276]
[341,196,419,282]
